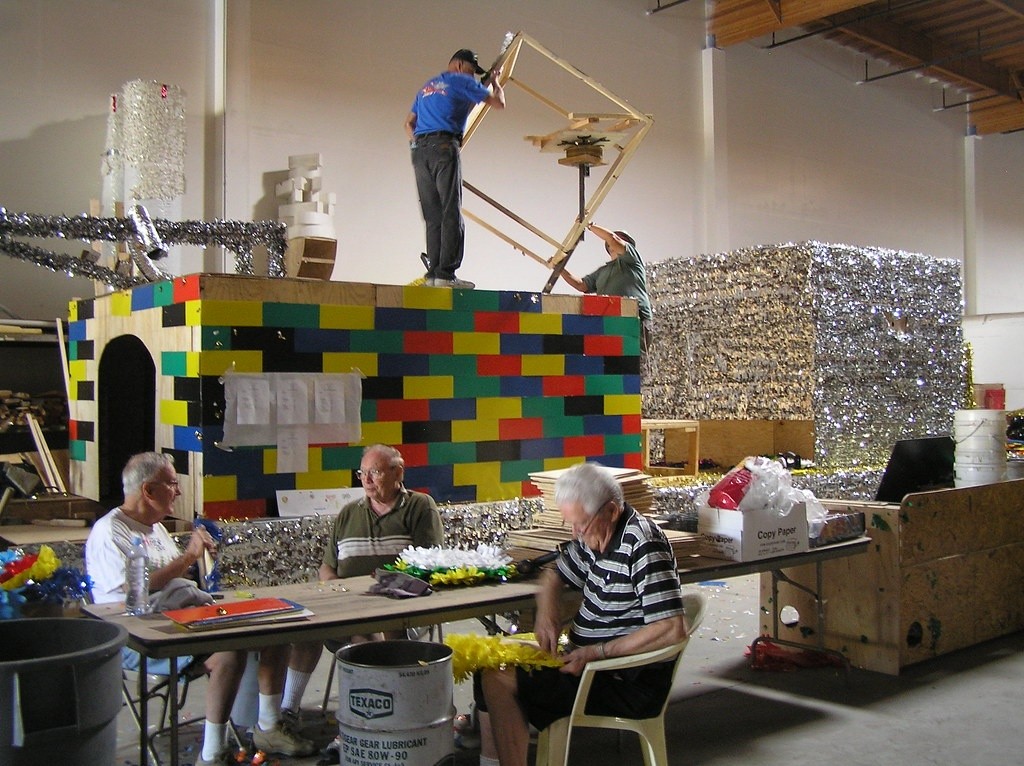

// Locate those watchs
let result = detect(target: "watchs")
[587,221,595,230]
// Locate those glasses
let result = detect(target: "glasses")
[141,481,178,490]
[561,499,611,537]
[355,465,396,479]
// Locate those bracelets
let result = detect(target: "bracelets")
[597,642,606,661]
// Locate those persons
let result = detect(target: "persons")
[242,443,444,743]
[547,213,652,354]
[84,452,321,766]
[405,48,506,289]
[472,461,689,766]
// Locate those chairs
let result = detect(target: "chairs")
[81,586,245,766]
[534,591,708,766]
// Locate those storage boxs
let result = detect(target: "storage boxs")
[699,501,809,563]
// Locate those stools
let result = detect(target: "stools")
[321,621,442,713]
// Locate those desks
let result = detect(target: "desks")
[83,531,872,766]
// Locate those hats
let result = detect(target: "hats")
[605,230,636,255]
[453,48,485,75]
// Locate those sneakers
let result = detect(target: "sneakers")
[321,737,341,760]
[254,721,318,758]
[426,278,475,290]
[195,749,241,766]
[245,707,305,736]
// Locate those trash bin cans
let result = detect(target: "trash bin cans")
[334,639,457,766]
[1,619,130,766]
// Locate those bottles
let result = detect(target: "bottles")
[124,537,150,614]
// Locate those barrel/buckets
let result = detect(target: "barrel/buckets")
[949,409,1008,488]
[0,618,128,766]
[335,640,457,766]
